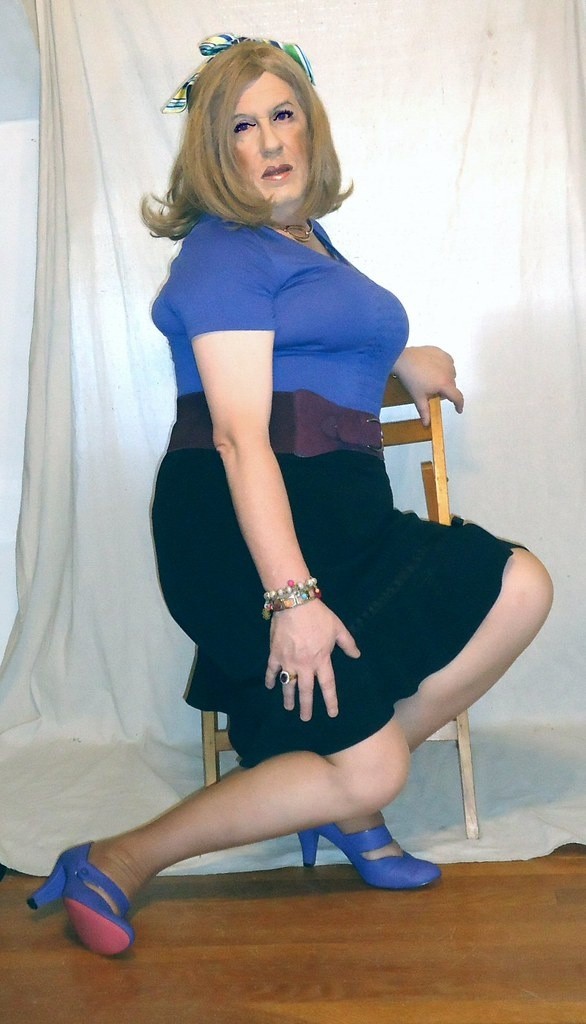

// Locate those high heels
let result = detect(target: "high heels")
[26,841,135,956]
[297,823,442,890]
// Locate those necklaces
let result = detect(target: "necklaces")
[270,220,315,242]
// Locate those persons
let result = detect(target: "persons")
[23,34,551,959]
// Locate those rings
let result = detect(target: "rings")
[279,670,297,685]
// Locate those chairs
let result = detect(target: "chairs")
[200,373,477,841]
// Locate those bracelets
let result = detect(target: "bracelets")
[262,577,322,621]
